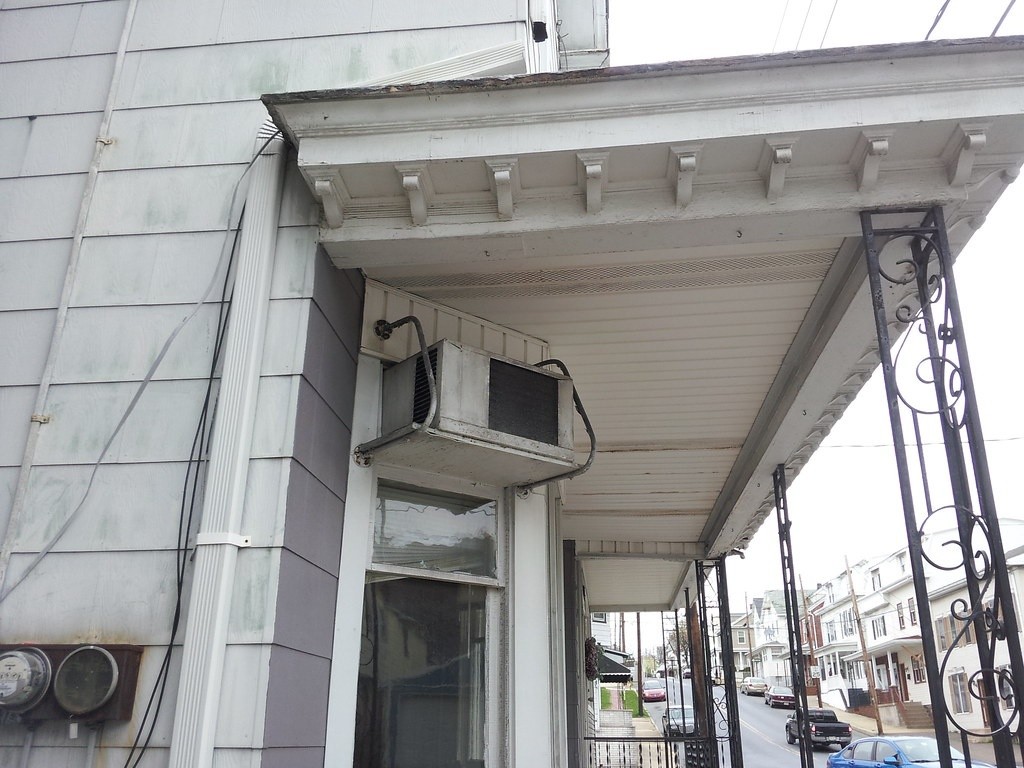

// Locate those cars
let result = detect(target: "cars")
[662,706,698,738]
[642,681,666,702]
[740,677,769,696]
[764,685,799,710]
[683,669,692,679]
[826,736,997,768]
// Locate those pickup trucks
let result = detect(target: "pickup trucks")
[785,708,853,749]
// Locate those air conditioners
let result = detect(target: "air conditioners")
[379,335,577,462]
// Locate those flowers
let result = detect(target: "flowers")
[586,636,604,683]
